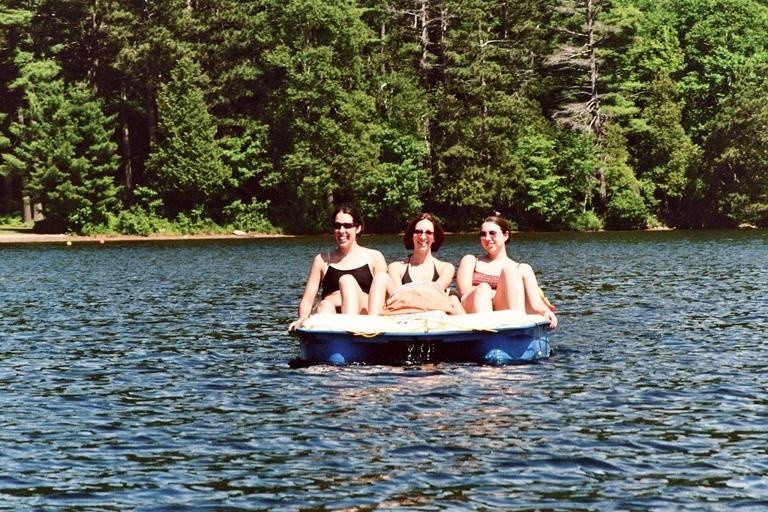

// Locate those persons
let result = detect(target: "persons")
[454,215,558,331]
[286,206,389,333]
[364,213,457,320]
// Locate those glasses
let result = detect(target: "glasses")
[414,229,435,235]
[479,231,499,236]
[333,222,357,229]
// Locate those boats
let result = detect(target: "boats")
[291,310,551,365]
